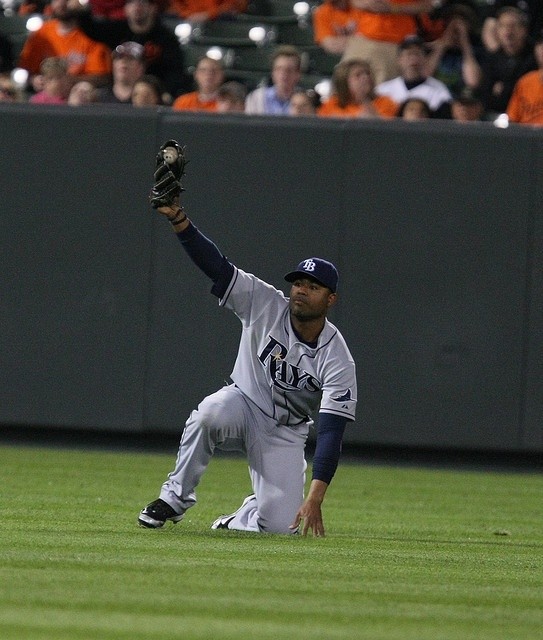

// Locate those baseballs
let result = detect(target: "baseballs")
[163,146,178,163]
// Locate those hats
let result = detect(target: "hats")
[283,256,338,293]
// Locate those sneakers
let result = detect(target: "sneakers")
[138,498,183,527]
[209,493,256,530]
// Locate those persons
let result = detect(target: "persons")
[166,0,248,31]
[285,90,322,117]
[245,46,304,117]
[0,0,185,109]
[137,139,358,541]
[315,0,543,127]
[170,54,227,116]
[214,83,245,116]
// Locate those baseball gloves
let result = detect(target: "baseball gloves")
[150,140,191,208]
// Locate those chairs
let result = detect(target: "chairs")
[71,15,130,44]
[0,13,50,65]
[143,1,345,98]
